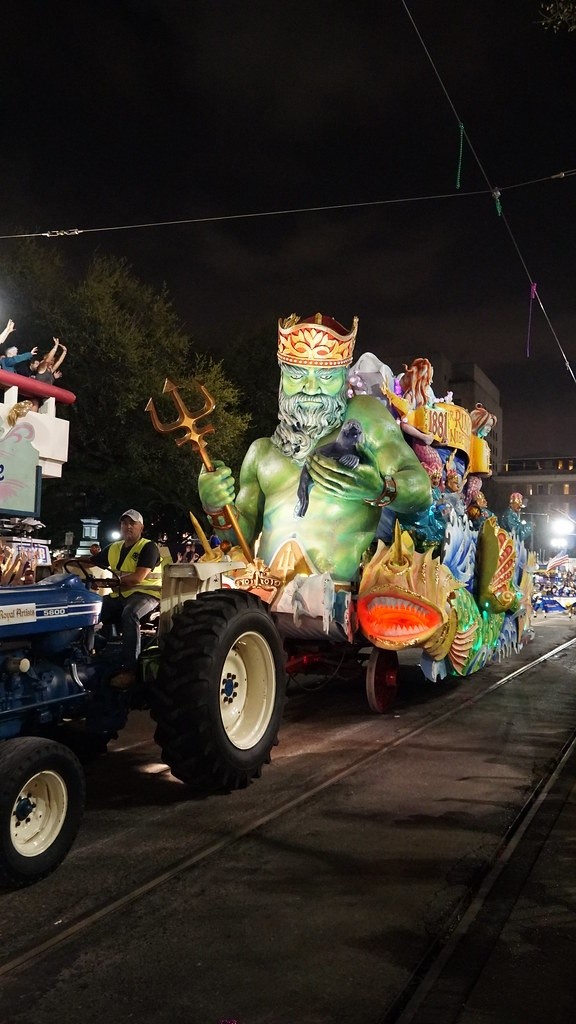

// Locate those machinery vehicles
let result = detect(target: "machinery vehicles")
[0,557,399,885]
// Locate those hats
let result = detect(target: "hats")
[120,508,144,524]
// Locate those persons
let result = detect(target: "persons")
[175,544,201,563]
[32,336,68,412]
[17,360,40,379]
[53,370,63,380]
[0,319,16,345]
[197,312,533,594]
[88,543,101,559]
[533,571,576,598]
[51,509,164,688]
[0,541,40,587]
[0,346,38,373]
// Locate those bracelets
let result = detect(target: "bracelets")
[364,476,398,508]
[204,505,239,531]
